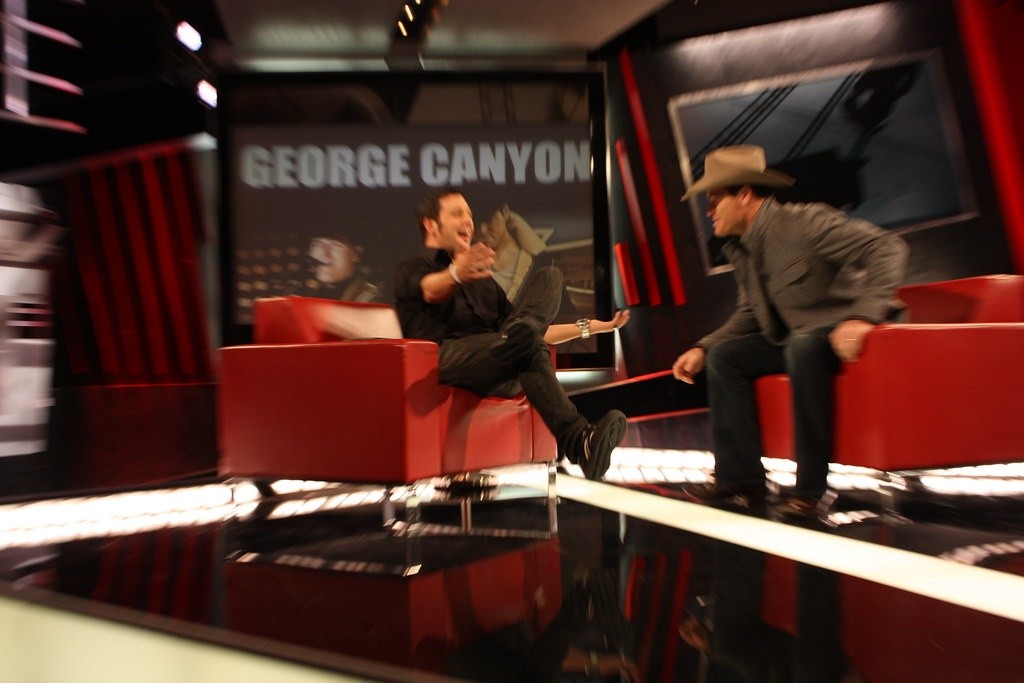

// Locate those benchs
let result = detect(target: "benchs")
[214,297,565,576]
[756,274,1024,528]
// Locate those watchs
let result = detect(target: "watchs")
[576,317,590,339]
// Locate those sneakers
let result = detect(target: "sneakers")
[488,318,540,366]
[578,409,627,481]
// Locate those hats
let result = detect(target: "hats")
[680,143,796,204]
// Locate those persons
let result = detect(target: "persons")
[458,179,575,323]
[671,144,910,522]
[394,187,631,479]
[284,205,392,305]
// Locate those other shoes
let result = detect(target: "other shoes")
[680,482,768,512]
[763,494,816,522]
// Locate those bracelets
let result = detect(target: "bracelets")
[448,264,464,285]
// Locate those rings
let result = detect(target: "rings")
[614,325,618,329]
[845,338,857,341]
